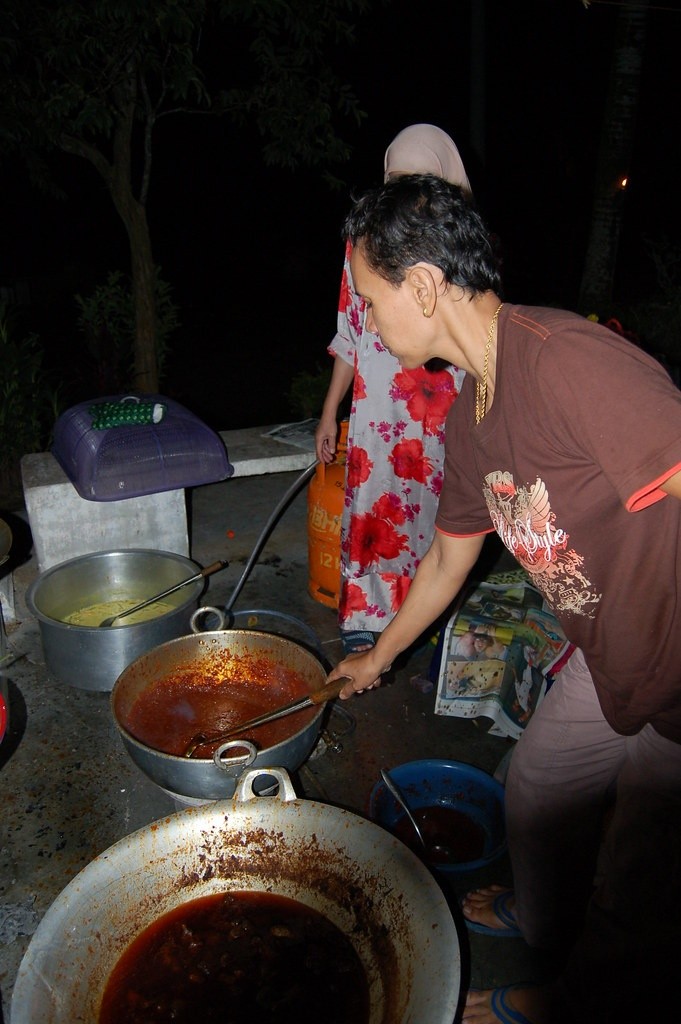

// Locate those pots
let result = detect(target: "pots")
[11,767,461,1024]
[110,607,331,801]
[25,548,204,692]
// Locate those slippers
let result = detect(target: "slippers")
[339,627,375,654]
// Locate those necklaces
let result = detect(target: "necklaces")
[475,303,504,424]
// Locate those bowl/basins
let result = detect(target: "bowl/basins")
[371,760,507,873]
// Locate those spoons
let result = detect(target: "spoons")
[378,768,449,859]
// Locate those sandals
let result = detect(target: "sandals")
[465,884,526,938]
[454,985,554,1024]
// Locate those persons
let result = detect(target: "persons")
[464,634,503,661]
[512,658,533,723]
[316,123,474,655]
[326,173,681,1024]
